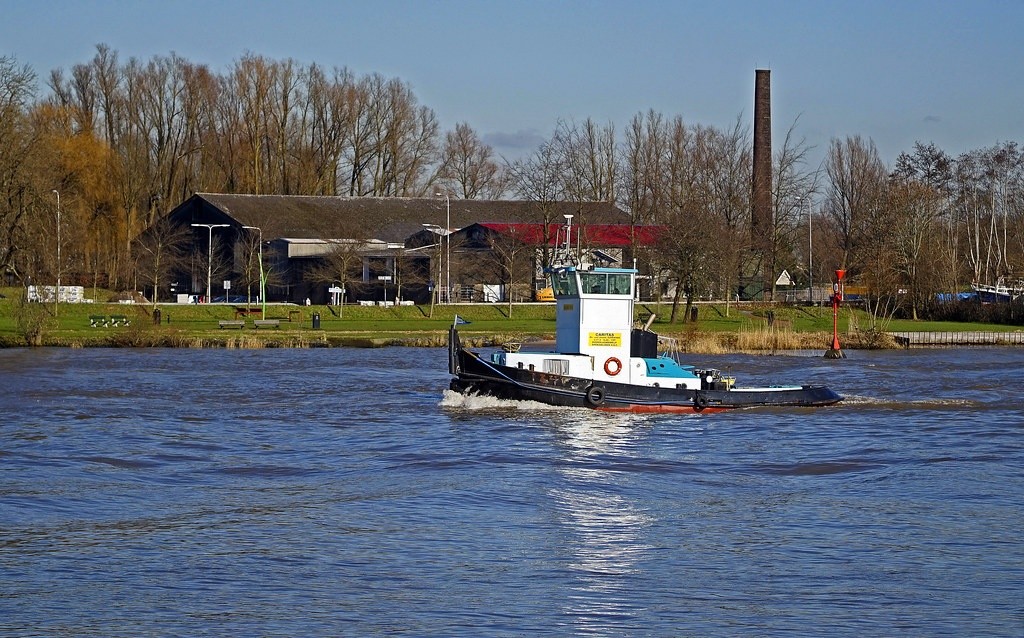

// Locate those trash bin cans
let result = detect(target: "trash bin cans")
[690,307,698,323]
[312,311,320,329]
[767,309,775,326]
[680,365,696,375]
[153,309,162,325]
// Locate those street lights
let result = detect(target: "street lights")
[794,196,814,301]
[52,190,61,303]
[633,257,637,296]
[191,224,230,303]
[242,226,262,301]
[436,193,450,303]
[422,224,442,303]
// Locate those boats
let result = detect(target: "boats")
[449,266,845,413]
[971,276,1024,302]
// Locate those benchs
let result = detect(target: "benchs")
[89,316,109,327]
[254,320,279,329]
[638,312,664,321]
[109,315,131,327]
[234,307,263,320]
[219,320,245,329]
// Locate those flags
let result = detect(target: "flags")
[457,315,472,325]
